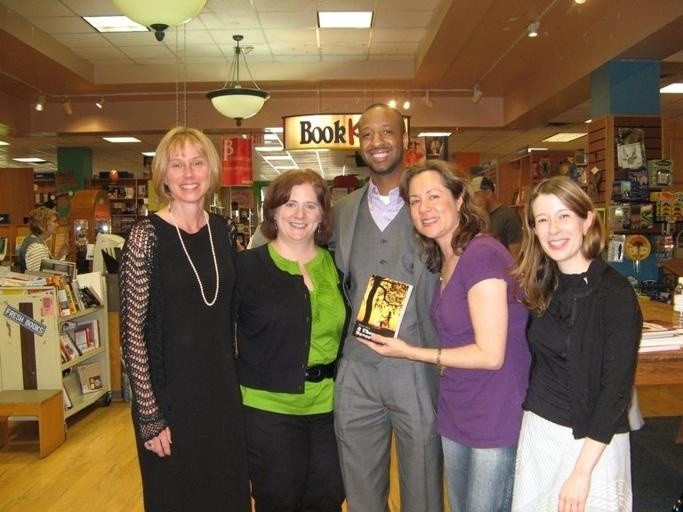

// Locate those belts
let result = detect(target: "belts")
[305,362,334,382]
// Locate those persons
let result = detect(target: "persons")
[118,125,252,511]
[357,157,532,512]
[232,167,346,511]
[19,205,72,274]
[248,103,445,512]
[468,175,524,262]
[507,174,646,512]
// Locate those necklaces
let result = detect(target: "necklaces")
[165,201,220,309]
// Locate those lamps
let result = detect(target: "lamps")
[33,90,106,116]
[112,1,207,40]
[528,20,542,39]
[422,88,483,109]
[208,36,271,125]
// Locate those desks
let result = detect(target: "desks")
[635,301,683,444]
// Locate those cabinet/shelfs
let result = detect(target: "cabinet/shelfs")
[32,178,160,234]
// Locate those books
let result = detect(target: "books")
[209,186,258,251]
[637,319,683,353]
[99,180,149,233]
[0,273,110,407]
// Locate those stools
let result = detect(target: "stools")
[0,390,65,458]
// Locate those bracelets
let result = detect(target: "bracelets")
[432,347,448,377]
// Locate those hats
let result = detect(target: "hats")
[471,176,495,193]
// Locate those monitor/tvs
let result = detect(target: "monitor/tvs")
[93,232,125,271]
